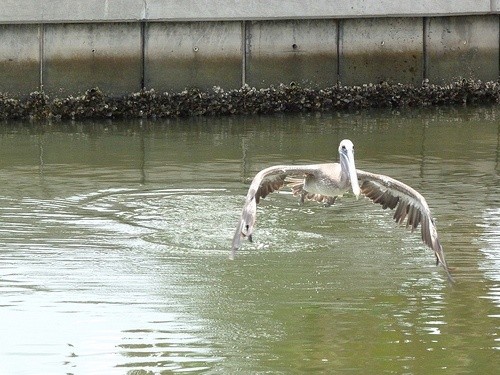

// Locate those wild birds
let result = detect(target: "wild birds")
[228,139,454,283]
